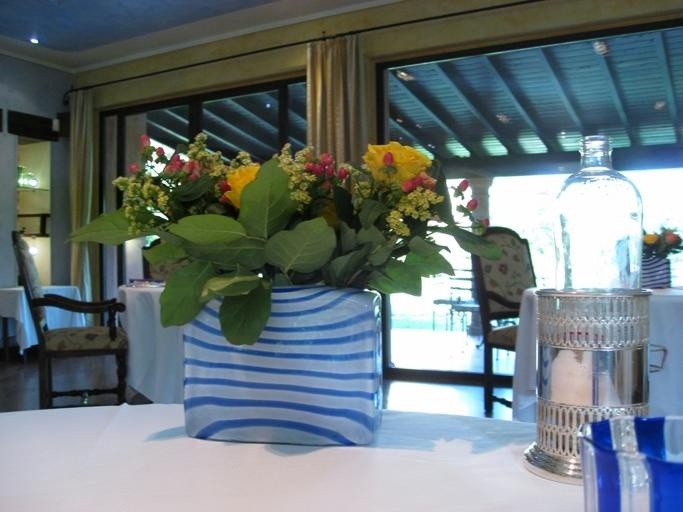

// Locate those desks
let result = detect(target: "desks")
[0,404,585,512]
[5,280,186,407]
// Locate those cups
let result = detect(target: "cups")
[577,415,683,512]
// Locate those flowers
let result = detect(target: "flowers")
[67,129,506,346]
[636,224,683,258]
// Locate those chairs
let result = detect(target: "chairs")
[13,230,129,407]
[471,226,541,417]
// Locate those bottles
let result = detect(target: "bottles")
[556,135,644,293]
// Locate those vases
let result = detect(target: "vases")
[641,258,674,288]
[179,274,388,446]
